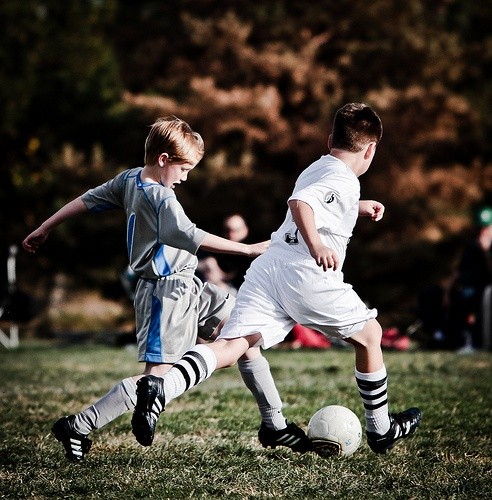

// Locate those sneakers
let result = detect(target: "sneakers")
[51,415,92,463]
[366,407,421,454]
[131,375,166,447]
[258,419,318,455]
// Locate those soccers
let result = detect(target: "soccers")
[306,405,363,460]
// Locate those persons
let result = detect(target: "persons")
[23,114,312,467]
[0,208,492,356]
[129,102,422,455]
